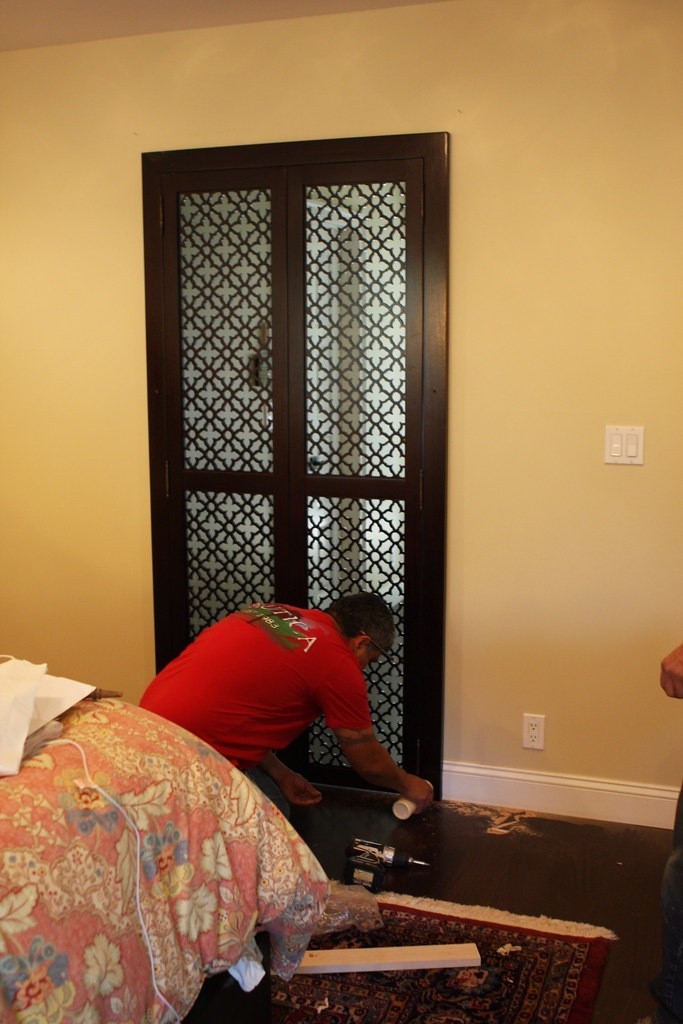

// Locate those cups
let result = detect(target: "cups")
[392,780,433,820]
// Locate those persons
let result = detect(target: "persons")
[658,643,683,1024]
[137,591,434,821]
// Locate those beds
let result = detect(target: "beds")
[0,652,331,1024]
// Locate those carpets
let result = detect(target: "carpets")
[249,885,620,1023]
